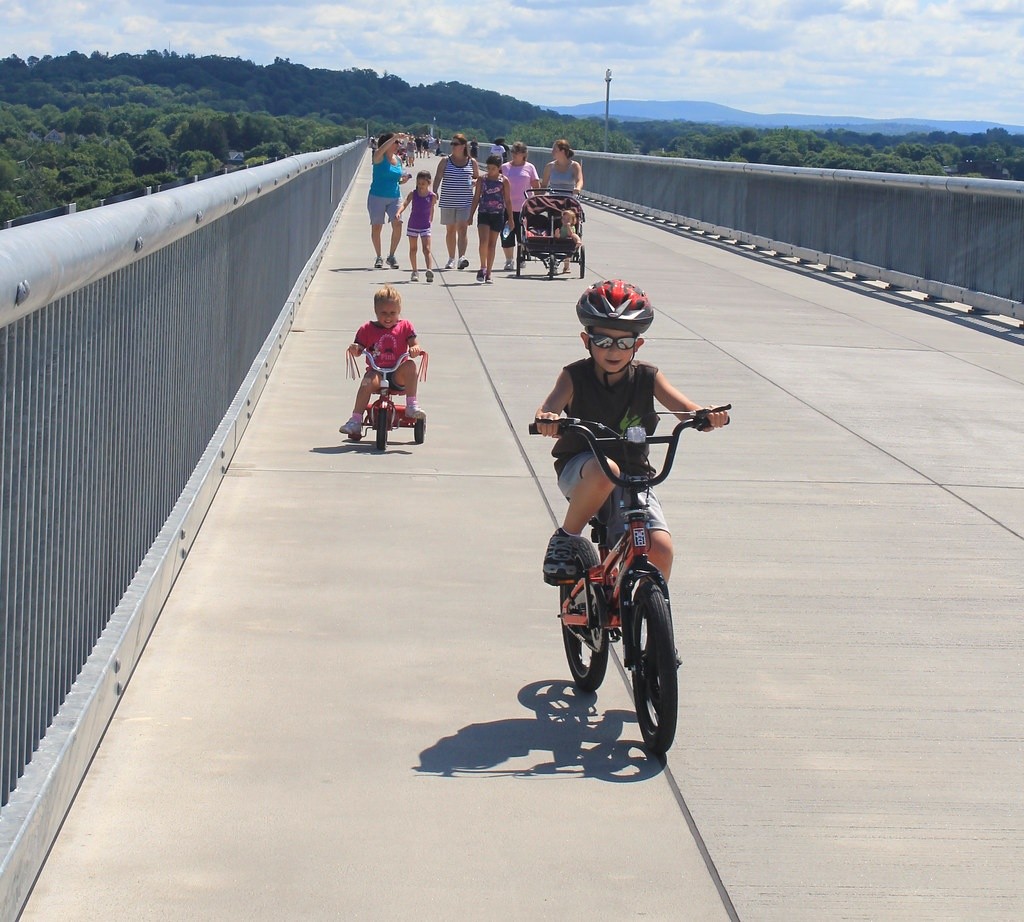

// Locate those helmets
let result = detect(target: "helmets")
[576,278,654,334]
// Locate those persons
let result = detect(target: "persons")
[541,139,583,272]
[469,139,479,158]
[536,280,728,665]
[368,132,410,267]
[395,170,437,282]
[339,289,426,436]
[397,132,441,167]
[500,141,540,270]
[560,209,577,238]
[491,138,510,163]
[434,134,479,270]
[370,135,378,164]
[469,154,515,285]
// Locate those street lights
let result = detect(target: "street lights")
[603,68,612,151]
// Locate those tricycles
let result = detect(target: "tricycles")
[347,346,429,451]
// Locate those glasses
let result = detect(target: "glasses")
[394,140,399,144]
[586,332,638,350]
[450,142,462,147]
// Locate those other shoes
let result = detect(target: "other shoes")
[411,271,419,281]
[404,161,415,167]
[563,267,571,273]
[426,269,434,282]
[547,269,557,275]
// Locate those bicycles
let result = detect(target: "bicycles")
[528,403,732,754]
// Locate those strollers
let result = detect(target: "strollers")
[516,188,587,282]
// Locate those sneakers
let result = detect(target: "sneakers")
[476,269,487,281]
[641,648,683,670]
[520,260,526,267]
[543,528,578,579]
[457,255,469,269]
[485,272,493,284]
[405,404,426,419]
[445,258,455,268]
[339,417,361,434]
[374,255,383,268]
[386,255,399,269]
[504,259,514,271]
